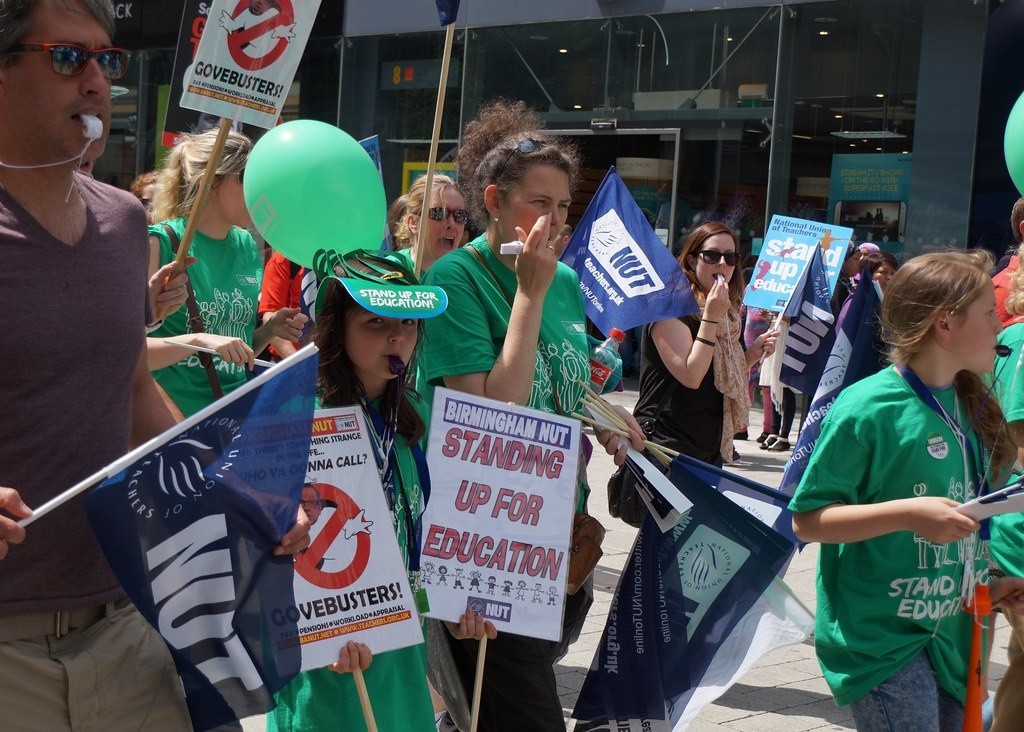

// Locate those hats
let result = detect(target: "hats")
[313,246,447,327]
[858,242,879,256]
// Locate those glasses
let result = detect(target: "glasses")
[219,167,243,184]
[693,249,741,267]
[496,138,557,180]
[413,207,470,224]
[0,43,130,80]
[299,499,321,508]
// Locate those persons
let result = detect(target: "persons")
[739,241,894,451]
[261,253,496,732]
[965,199,1024,732]
[416,109,646,732]
[790,258,1024,732]
[132,128,319,421]
[300,483,321,524]
[385,175,469,279]
[0,1,312,732]
[635,224,782,468]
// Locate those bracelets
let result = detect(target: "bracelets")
[696,336,715,346]
[701,319,719,324]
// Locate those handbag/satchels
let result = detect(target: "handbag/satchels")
[565,513,606,596]
[608,464,645,528]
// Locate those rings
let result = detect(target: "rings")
[548,246,555,252]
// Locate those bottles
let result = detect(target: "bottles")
[586,328,626,396]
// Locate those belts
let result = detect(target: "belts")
[0,596,132,644]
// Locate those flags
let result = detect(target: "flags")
[783,244,837,397]
[776,273,886,552]
[85,353,317,732]
[559,172,700,335]
[572,455,816,732]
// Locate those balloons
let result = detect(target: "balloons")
[243,120,386,272]
[1004,91,1024,196]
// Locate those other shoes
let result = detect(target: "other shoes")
[734,429,749,441]
[757,432,767,442]
[760,433,780,448]
[768,436,790,451]
